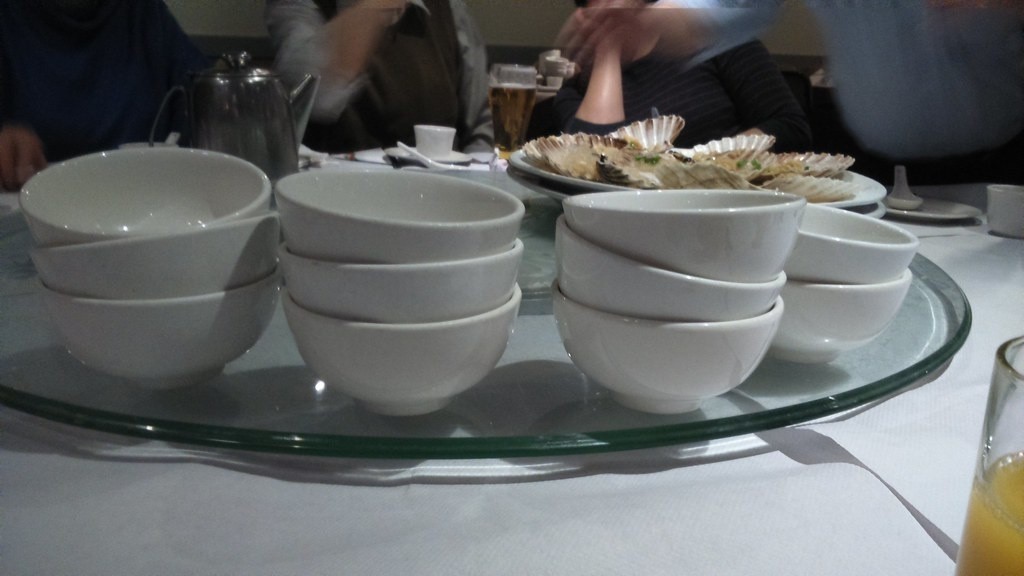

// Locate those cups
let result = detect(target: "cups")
[986,184,1022,239]
[413,126,457,160]
[955,334,1023,576]
[548,77,563,89]
[486,65,540,160]
[545,57,568,77]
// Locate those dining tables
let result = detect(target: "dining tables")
[0,173,1024,576]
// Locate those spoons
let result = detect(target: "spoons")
[887,162,924,209]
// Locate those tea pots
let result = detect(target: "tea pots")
[150,50,319,198]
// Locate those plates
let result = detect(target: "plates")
[888,199,983,221]
[353,148,474,165]
[537,86,562,92]
[506,149,887,220]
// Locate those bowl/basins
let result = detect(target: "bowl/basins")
[18,142,525,416]
[553,189,919,414]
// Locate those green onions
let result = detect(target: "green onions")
[632,154,764,171]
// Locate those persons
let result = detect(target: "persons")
[0,0,211,190]
[525,0,1024,189]
[262,0,496,155]
